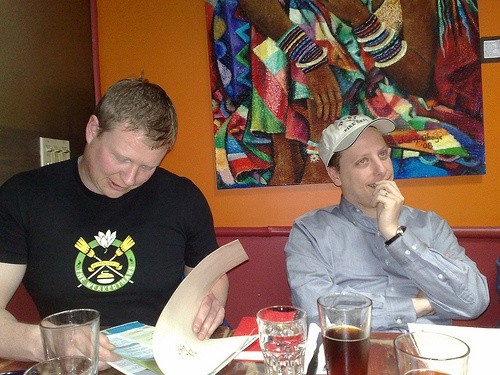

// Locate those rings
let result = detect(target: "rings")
[386,192,389,196]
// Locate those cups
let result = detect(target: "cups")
[39,308,100,375]
[23,355,95,375]
[393,330,471,375]
[256,305,308,375]
[317,292,373,375]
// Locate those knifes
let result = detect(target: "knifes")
[306,331,322,375]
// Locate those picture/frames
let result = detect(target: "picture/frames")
[480,36,500,63]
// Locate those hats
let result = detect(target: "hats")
[319,114,397,168]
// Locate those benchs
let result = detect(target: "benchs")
[212,227,500,330]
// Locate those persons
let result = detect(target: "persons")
[284,115,490,332]
[0,78,228,372]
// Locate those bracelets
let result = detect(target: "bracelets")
[385,226,407,246]
